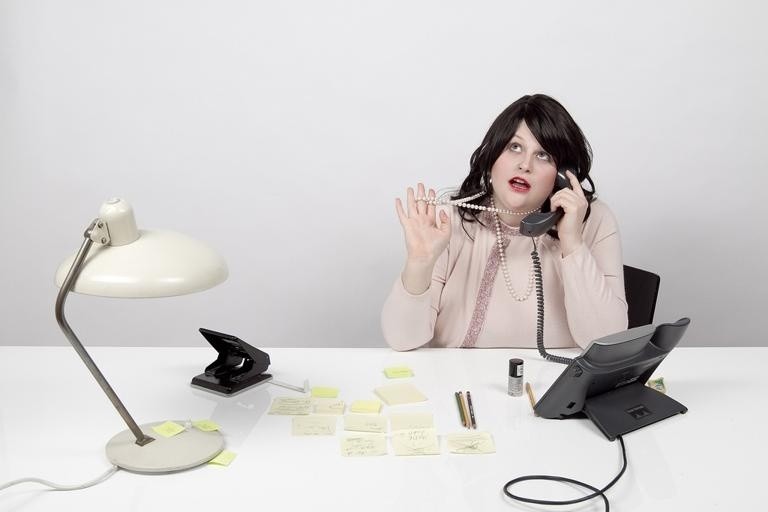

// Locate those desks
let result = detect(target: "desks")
[1,346,764,510]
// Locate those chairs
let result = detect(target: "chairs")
[619,262,659,333]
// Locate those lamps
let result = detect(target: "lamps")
[50,196,229,498]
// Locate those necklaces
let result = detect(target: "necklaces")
[411,182,547,302]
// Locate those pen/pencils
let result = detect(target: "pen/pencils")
[455,391,476,429]
[526,382,539,416]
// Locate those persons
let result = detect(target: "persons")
[380,93,630,352]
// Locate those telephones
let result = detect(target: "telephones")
[520,164,577,236]
